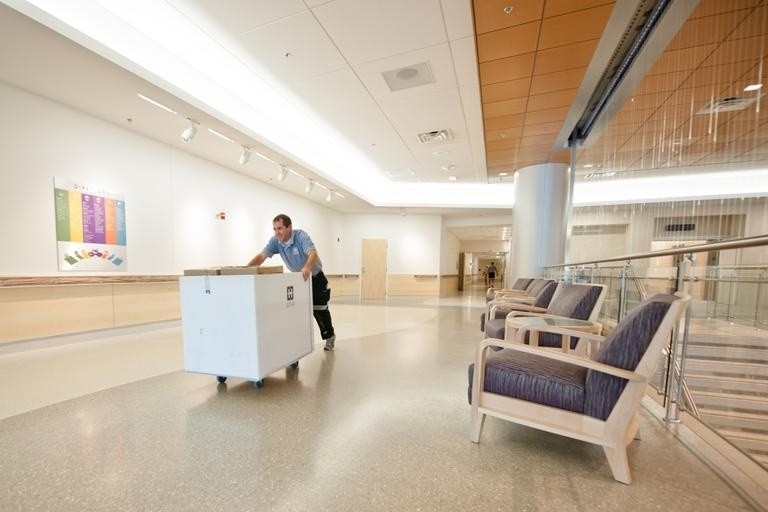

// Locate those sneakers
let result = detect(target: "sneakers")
[324,335,335,350]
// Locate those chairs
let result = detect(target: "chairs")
[468,289,693,484]
[478,279,610,353]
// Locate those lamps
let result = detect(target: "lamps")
[138,93,345,202]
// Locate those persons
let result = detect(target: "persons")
[247,214,335,351]
[479,262,498,288]
